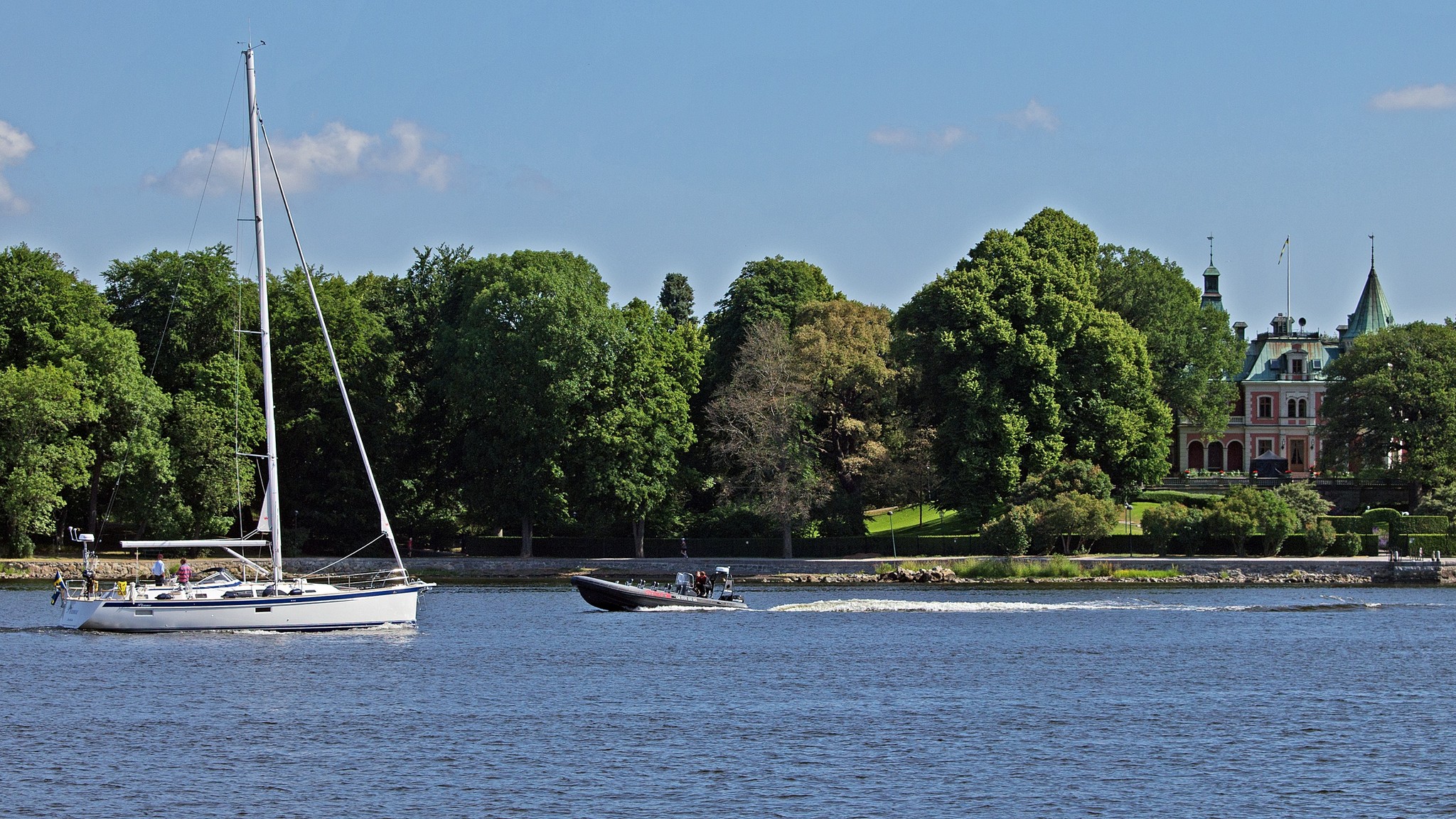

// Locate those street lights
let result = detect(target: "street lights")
[887,511,896,559]
[1126,505,1133,557]
[295,510,299,559]
[1124,500,1129,535]
[939,509,946,556]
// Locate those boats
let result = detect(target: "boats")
[572,567,748,612]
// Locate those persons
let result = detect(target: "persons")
[405,538,413,558]
[176,557,192,585]
[690,571,712,596]
[681,538,689,559]
[152,554,165,587]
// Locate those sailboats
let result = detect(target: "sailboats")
[51,16,439,631]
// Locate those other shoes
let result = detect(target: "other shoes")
[702,592,706,596]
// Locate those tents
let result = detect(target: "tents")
[1250,450,1289,478]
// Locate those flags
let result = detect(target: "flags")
[51,572,66,604]
